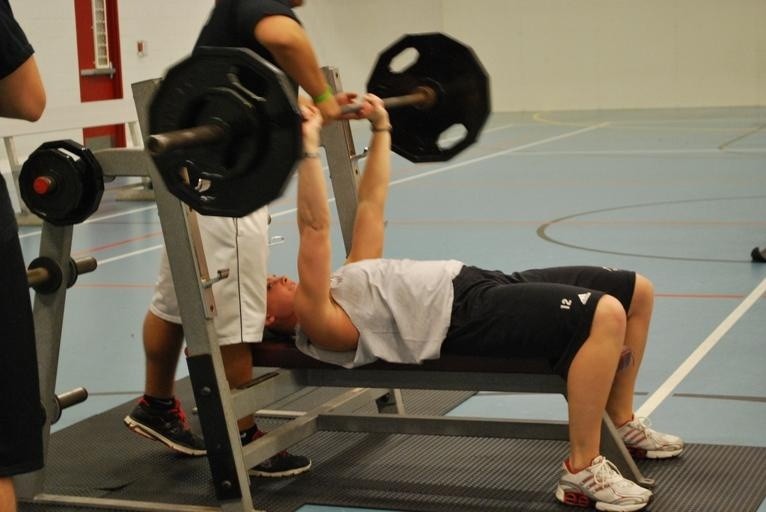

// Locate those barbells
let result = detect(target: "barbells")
[147,33,492,218]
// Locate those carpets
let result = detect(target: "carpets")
[1,372,766,512]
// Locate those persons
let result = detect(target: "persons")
[256,93,688,511]
[119,1,344,481]
[0,1,47,512]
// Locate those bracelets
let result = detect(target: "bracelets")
[313,84,334,104]
[301,149,323,159]
[371,124,394,132]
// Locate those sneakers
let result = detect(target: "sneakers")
[124,401,205,456]
[242,432,313,478]
[554,456,655,512]
[616,414,684,458]
